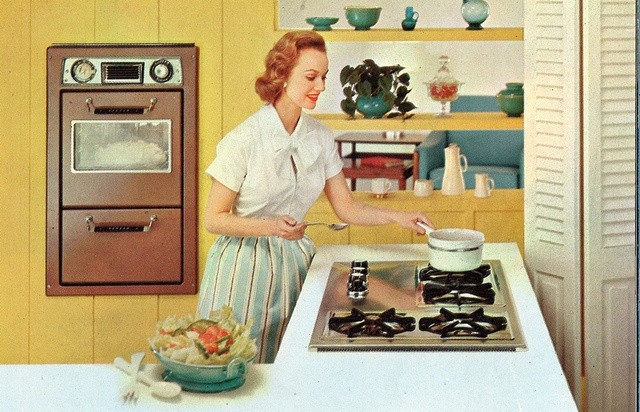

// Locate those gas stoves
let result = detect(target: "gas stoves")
[308,259,527,351]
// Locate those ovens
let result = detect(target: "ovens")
[46,46,198,296]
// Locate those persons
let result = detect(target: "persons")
[195,31,436,363]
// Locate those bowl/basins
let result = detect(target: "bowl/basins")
[149,344,258,384]
[343,6,383,31]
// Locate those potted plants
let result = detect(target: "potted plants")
[339,59,418,123]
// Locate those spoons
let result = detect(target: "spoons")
[296,221,348,230]
[113,356,181,398]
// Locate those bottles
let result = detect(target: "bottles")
[441,143,468,195]
[473,173,495,198]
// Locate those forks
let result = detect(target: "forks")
[120,352,146,399]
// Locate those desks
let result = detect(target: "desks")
[334,132,424,159]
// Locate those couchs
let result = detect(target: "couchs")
[412,96,525,190]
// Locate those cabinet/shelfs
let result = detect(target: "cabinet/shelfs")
[300,208,525,258]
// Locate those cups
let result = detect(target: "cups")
[371,178,392,194]
[414,179,434,196]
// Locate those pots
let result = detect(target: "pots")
[417,221,485,273]
